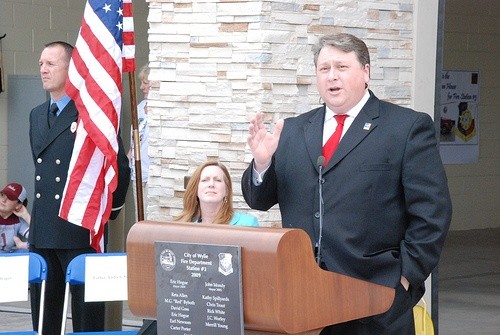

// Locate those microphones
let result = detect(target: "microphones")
[317,156,325,267]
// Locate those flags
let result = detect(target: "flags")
[58,0,137,255]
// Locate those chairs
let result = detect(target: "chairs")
[0,252,139,335]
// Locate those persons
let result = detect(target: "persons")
[240,32,453,335]
[0,183,31,253]
[125,63,150,225]
[167,161,261,228]
[26,40,131,333]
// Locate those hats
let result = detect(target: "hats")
[0,182,27,203]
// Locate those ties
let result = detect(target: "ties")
[48,103,59,128]
[322,115,349,169]
